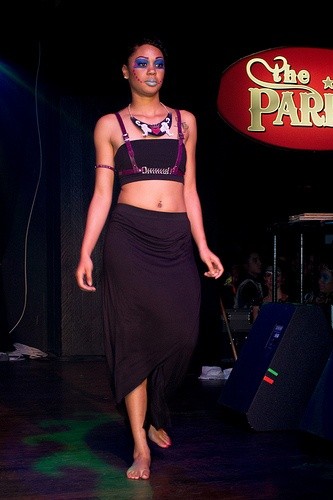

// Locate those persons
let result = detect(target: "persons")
[225,249,333,350]
[74,35,225,480]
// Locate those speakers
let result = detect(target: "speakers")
[217,302,333,441]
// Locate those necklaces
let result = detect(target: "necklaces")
[128,100,174,138]
